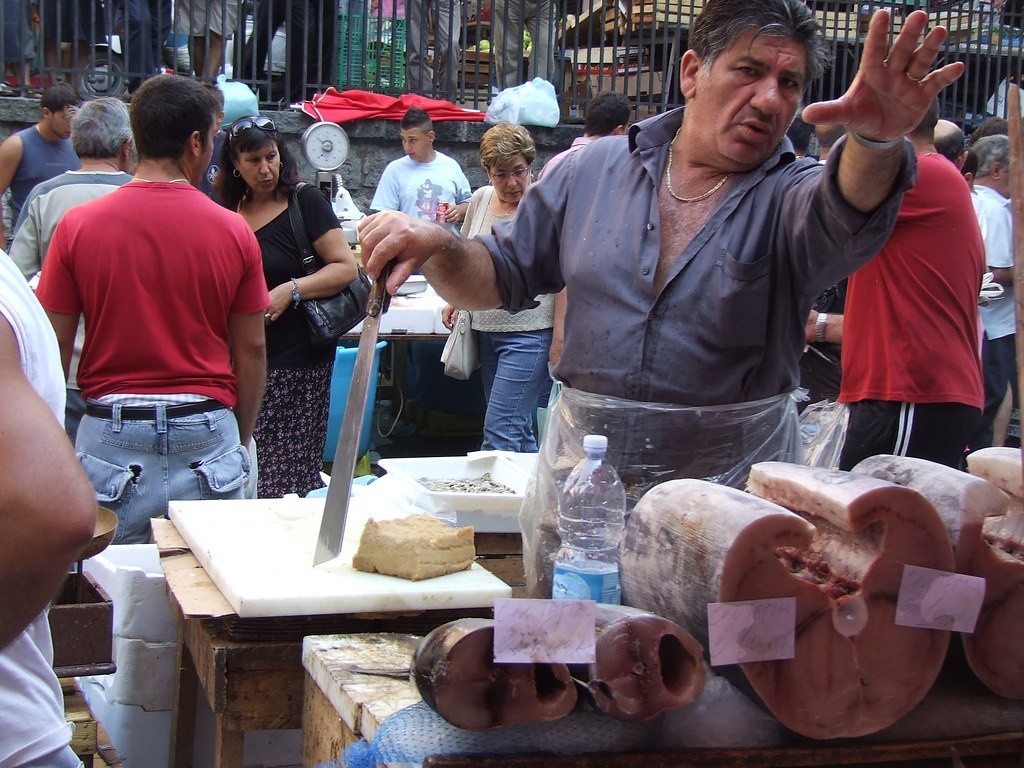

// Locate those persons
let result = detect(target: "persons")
[370,92,631,453]
[358,0,964,600]
[0,74,360,767]
[0,0,557,107]
[786,108,1018,473]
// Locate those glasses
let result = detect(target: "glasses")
[230,116,277,143]
[488,165,532,182]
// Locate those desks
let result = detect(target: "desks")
[169,532,530,768]
[302,634,1024,768]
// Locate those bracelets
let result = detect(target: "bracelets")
[850,132,904,149]
[291,278,300,306]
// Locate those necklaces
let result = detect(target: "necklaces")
[500,199,517,214]
[666,128,734,203]
[815,313,827,342]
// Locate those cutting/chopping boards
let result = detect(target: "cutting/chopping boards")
[166,495,513,618]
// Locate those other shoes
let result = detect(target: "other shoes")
[25,88,43,98]
[2,84,21,96]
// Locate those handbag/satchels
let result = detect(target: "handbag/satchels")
[288,182,372,345]
[440,312,480,381]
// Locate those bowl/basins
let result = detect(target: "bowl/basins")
[396,275,430,295]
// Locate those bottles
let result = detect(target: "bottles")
[553,434,628,606]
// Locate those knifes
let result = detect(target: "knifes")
[314,258,397,570]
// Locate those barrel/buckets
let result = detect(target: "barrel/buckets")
[322,340,388,461]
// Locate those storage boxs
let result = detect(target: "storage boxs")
[377,456,530,533]
[563,72,663,122]
[986,77,1024,121]
[345,284,454,335]
[75,544,301,768]
[599,0,1024,48]
[336,15,406,93]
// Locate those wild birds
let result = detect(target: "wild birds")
[466,30,532,54]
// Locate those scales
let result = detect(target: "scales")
[301,122,358,247]
[47,506,118,678]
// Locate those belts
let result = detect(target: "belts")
[83,397,228,421]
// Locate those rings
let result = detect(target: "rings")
[906,73,924,81]
[265,313,271,317]
